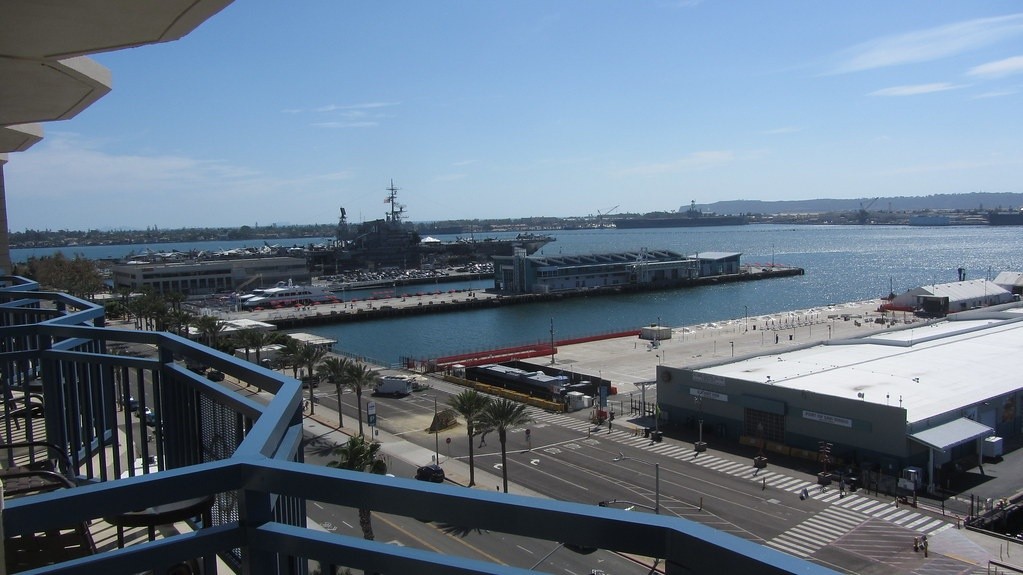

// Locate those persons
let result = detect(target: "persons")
[606,418,613,435]
[914,535,928,552]
[840,480,845,492]
[800,488,808,500]
[297,305,311,312]
[477,431,488,448]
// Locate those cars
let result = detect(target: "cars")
[120,393,164,437]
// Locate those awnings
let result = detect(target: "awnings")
[906,416,994,453]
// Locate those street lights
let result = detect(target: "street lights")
[420,393,439,466]
[612,453,660,564]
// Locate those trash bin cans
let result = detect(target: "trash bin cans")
[610,411,615,419]
[850,478,857,492]
[645,428,650,437]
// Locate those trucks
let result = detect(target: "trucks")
[373,375,413,398]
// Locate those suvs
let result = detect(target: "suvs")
[416,464,445,483]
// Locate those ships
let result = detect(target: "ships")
[280,177,558,276]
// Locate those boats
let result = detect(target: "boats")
[241,278,336,311]
